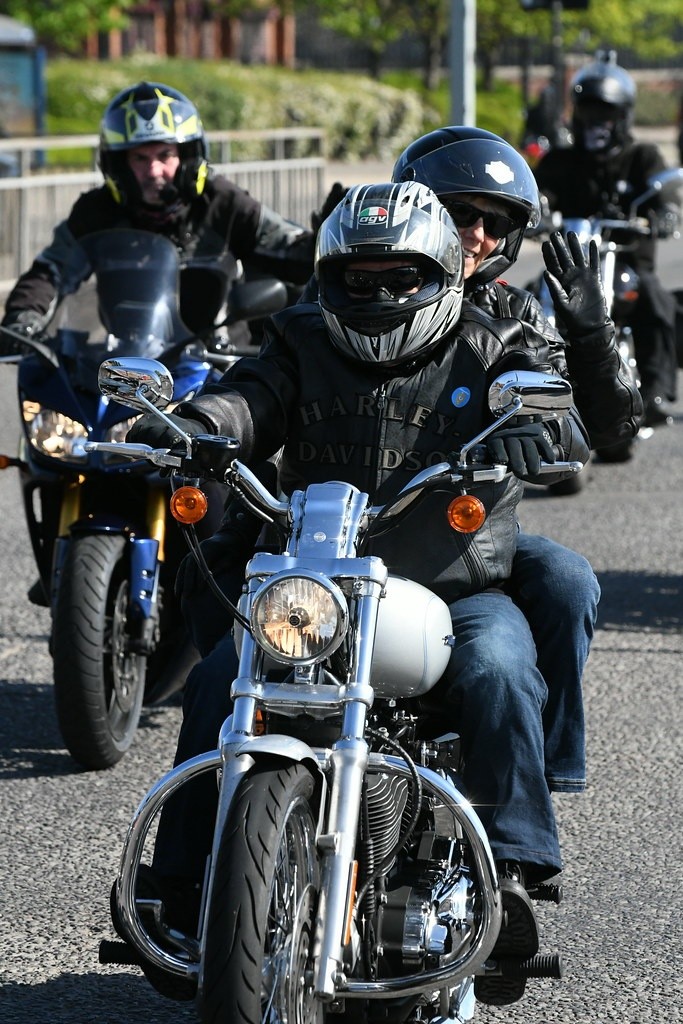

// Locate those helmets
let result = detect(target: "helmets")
[568,61,636,110]
[97,79,210,224]
[390,125,532,290]
[315,180,465,368]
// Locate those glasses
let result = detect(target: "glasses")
[337,264,427,299]
[436,197,521,241]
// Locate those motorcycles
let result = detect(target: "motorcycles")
[74,352,586,1024]
[1,228,292,769]
[521,167,683,494]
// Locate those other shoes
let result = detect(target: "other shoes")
[109,861,198,1000]
[641,385,673,426]
[473,863,540,1005]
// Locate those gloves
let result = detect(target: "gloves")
[125,411,213,480]
[1,310,50,365]
[644,210,675,240]
[309,181,354,244]
[541,230,617,349]
[475,416,556,478]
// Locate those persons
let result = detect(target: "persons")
[366,126,646,805]
[4,82,351,674]
[111,183,597,1011]
[526,69,682,425]
